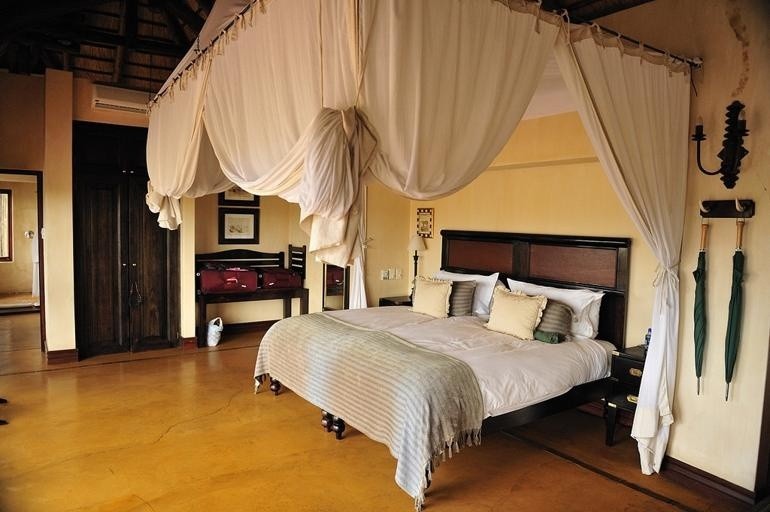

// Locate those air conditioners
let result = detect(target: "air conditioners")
[91,82,154,116]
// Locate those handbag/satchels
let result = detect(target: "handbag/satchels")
[207,316,225,347]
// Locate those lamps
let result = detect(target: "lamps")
[690,98,752,191]
[407,234,428,301]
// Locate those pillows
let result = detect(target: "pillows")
[406,270,607,344]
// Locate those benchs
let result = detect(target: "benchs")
[193,243,310,349]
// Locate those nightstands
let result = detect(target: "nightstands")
[603,340,649,447]
[378,295,412,307]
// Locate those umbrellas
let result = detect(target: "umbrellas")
[691,223,710,396]
[725,219,746,403]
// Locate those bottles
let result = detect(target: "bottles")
[644,328,652,351]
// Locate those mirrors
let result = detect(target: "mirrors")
[322,262,350,313]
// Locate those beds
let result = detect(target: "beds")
[251,228,633,512]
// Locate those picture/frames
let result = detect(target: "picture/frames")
[217,206,261,245]
[218,187,261,207]
[416,206,436,239]
